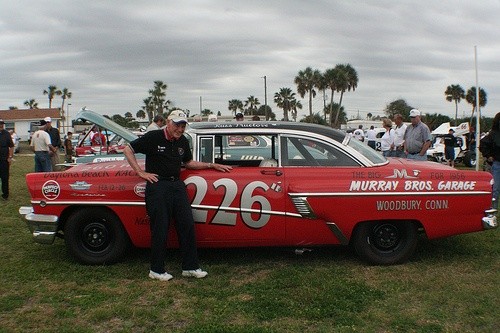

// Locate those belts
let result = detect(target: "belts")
[159,177,180,181]
[411,152,419,155]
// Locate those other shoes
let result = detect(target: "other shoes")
[149,270,173,280]
[182,268,208,278]
[482,216,498,227]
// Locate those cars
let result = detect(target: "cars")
[56,111,469,172]
[18,129,497,265]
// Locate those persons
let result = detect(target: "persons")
[193,113,261,122]
[353,109,432,161]
[0,117,107,200]
[440,112,500,202]
[122,110,234,281]
[145,115,163,134]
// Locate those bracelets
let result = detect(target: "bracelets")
[208,164,211,169]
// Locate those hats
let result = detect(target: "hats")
[44,117,52,122]
[169,110,189,126]
[450,129,455,133]
[0,119,6,124]
[393,114,403,119]
[409,109,420,117]
